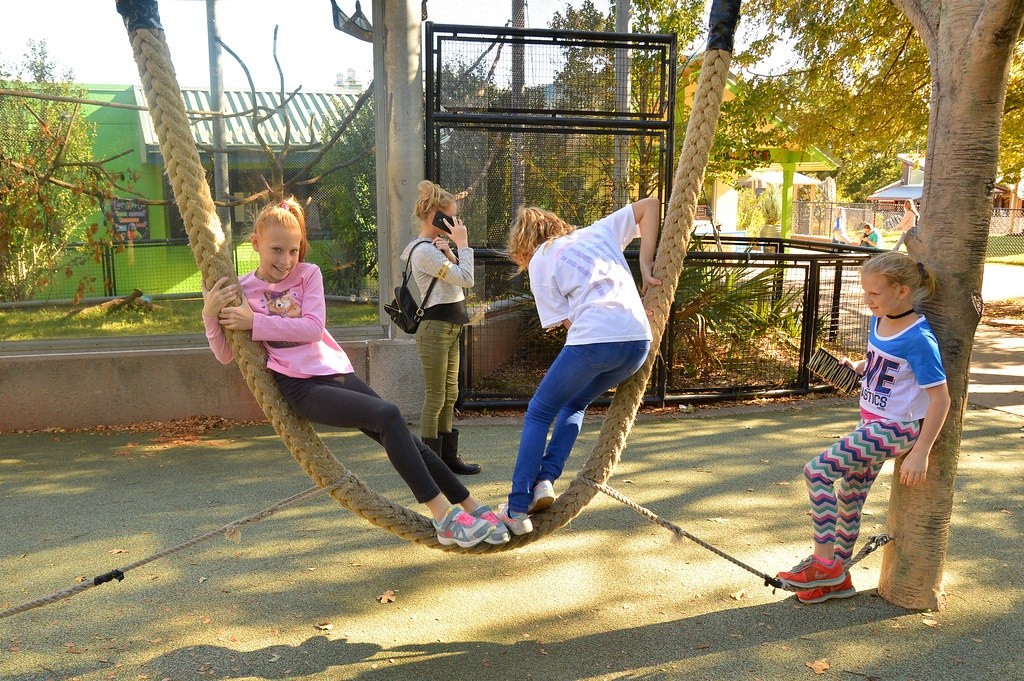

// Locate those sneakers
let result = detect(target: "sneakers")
[498,503,534,535]
[527,480,555,514]
[469,505,512,545]
[774,555,846,588]
[796,571,856,604]
[433,503,497,549]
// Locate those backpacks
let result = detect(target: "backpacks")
[384,240,437,334]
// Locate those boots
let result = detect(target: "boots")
[422,435,443,460]
[439,428,481,476]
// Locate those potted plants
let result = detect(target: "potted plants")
[759,190,784,256]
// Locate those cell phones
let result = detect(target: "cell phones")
[432,211,454,234]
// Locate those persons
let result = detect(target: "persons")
[497,195,665,538]
[857,224,878,250]
[198,197,514,548]
[397,179,484,482]
[891,201,920,253]
[827,206,848,256]
[773,252,952,608]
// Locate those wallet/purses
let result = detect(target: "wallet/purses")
[806,347,863,394]
